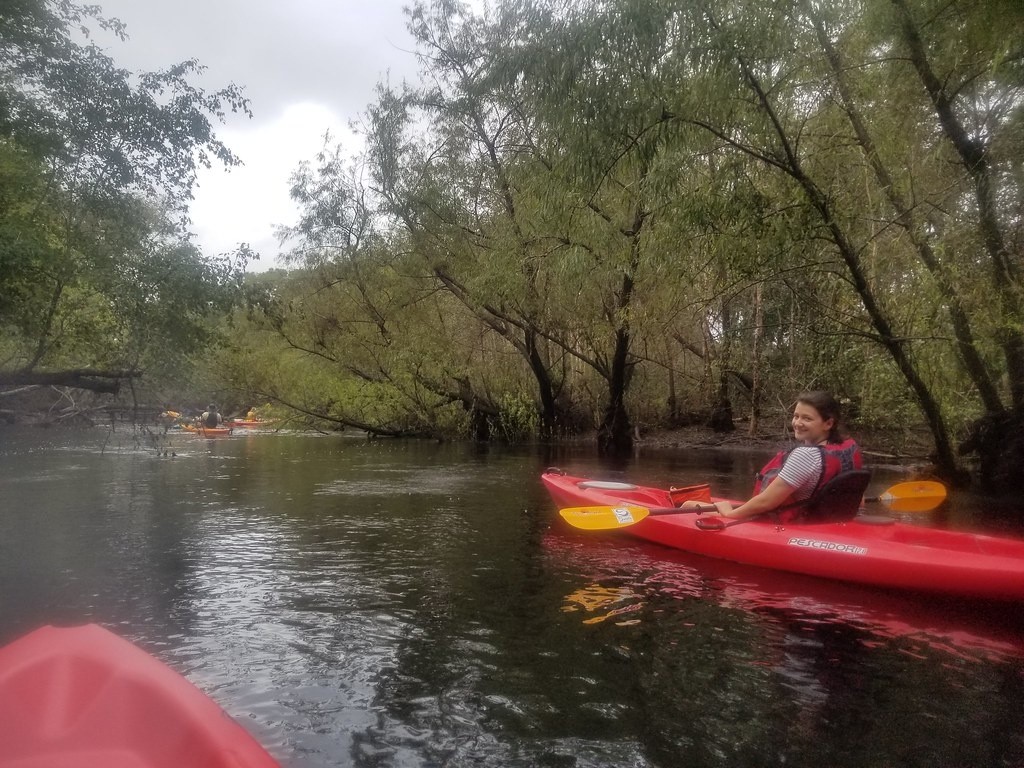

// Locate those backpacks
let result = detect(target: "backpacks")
[206,413,218,429]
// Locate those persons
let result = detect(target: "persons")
[196,402,222,429]
[713,390,864,523]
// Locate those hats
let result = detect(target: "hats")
[206,404,217,412]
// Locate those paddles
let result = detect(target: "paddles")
[556,478,945,532]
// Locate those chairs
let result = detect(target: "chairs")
[802,469,871,524]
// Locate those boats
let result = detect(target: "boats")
[542,466,1023,604]
[233,418,281,427]
[166,409,231,436]
